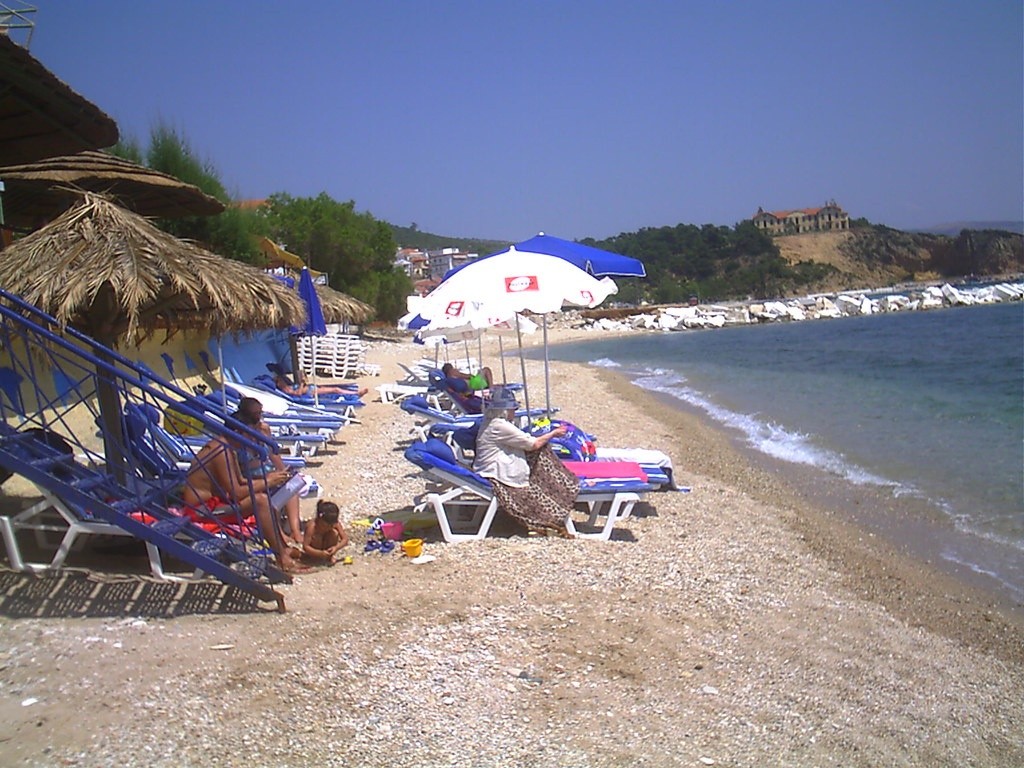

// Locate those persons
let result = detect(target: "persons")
[471,387,582,540]
[182,398,319,575]
[302,499,349,565]
[272,369,369,399]
[441,362,495,393]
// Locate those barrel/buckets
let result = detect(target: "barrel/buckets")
[382,521,422,557]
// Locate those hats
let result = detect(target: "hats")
[488,389,520,409]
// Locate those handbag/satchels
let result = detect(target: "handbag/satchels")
[470,373,488,390]
[268,470,307,512]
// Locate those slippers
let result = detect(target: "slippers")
[365,540,381,551]
[380,541,394,552]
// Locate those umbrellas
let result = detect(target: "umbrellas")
[395,229,648,437]
[287,265,329,411]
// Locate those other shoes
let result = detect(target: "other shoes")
[536,528,548,535]
[558,529,575,539]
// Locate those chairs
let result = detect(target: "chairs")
[296,334,381,379]
[373,354,692,543]
[0,374,366,583]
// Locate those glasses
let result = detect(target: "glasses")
[515,408,518,410]
